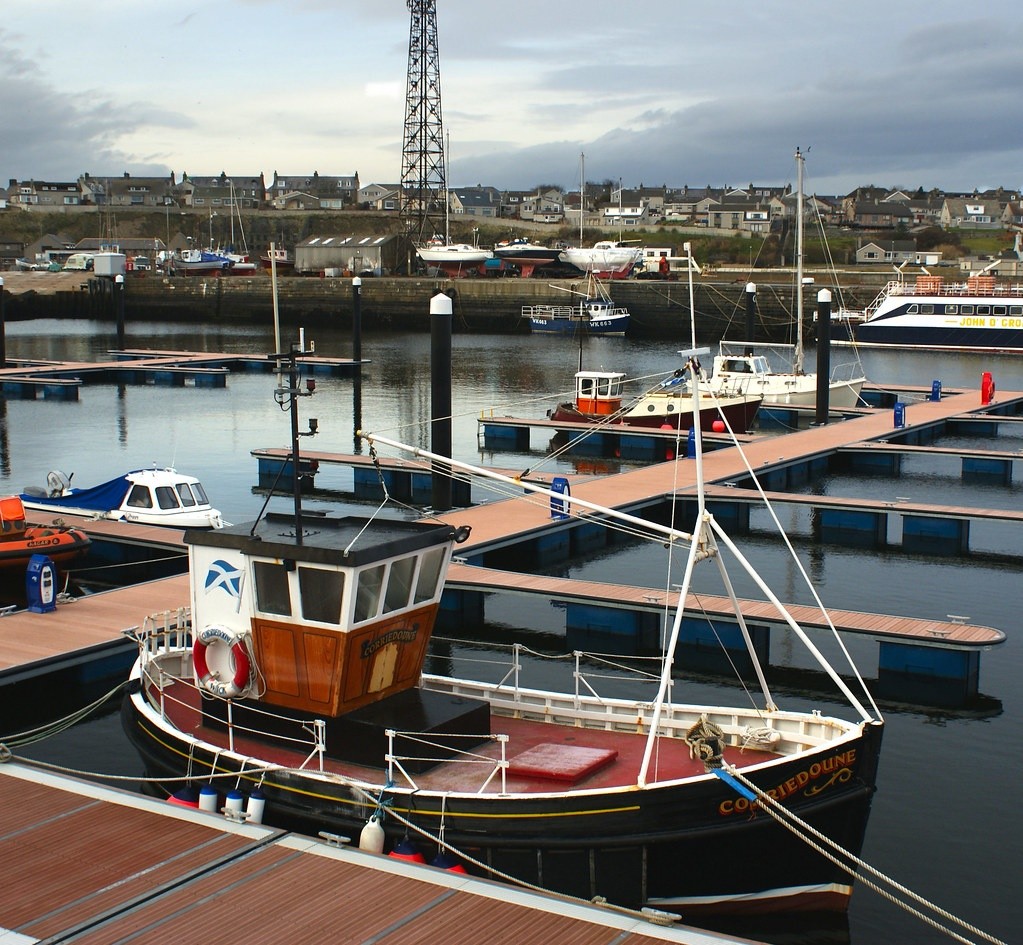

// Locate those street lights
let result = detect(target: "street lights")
[890,239,895,267]
[472,224,477,248]
[748,244,754,268]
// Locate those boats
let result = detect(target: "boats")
[544,297,766,448]
[519,254,632,338]
[259,230,296,278]
[415,151,644,281]
[1,493,96,583]
[13,461,226,532]
[115,242,896,911]
[813,255,1023,357]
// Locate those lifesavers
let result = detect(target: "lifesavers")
[193,624,249,698]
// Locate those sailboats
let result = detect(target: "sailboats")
[48,176,260,279]
[14,225,46,271]
[683,145,870,419]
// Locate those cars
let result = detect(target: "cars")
[29,260,58,271]
